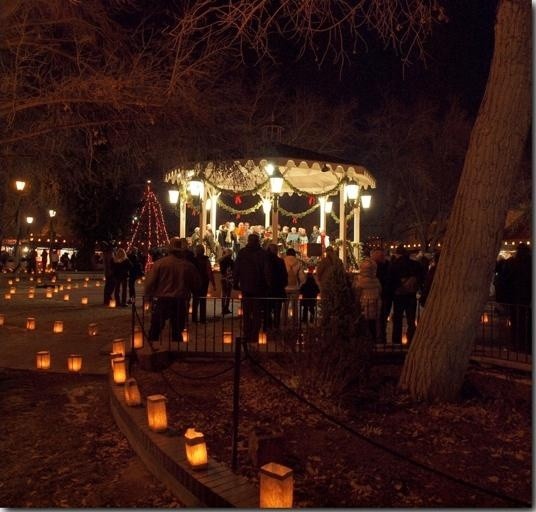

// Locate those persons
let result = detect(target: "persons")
[93,221,535,354]
[29,248,76,275]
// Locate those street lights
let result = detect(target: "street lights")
[16,181,57,270]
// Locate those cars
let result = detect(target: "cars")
[476,300,513,343]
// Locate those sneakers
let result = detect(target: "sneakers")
[191,309,234,323]
[113,299,136,309]
